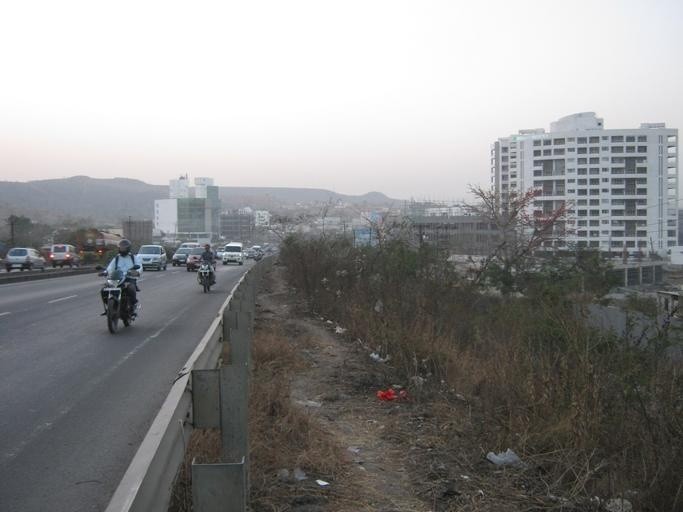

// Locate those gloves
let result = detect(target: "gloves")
[131,270,139,276]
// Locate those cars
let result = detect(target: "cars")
[5,248,47,271]
[172,242,223,269]
[137,245,168,271]
[245,246,265,260]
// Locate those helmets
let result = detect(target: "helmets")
[119,239,131,248]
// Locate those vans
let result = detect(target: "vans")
[51,244,80,267]
[223,242,247,264]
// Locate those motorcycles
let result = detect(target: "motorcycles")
[94,264,142,333]
[196,260,217,293]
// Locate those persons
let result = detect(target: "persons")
[198,243,216,284]
[100,239,144,317]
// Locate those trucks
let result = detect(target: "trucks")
[79,229,125,258]
